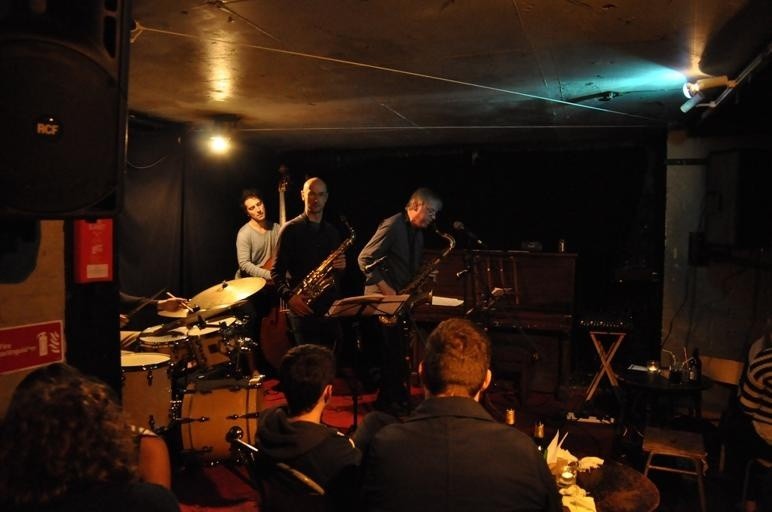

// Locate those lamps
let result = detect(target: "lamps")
[682,74,731,99]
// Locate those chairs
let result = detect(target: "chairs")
[231,439,327,512]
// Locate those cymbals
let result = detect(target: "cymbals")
[190,276,268,310]
[157,297,191,317]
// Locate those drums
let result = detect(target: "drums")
[187,314,248,370]
[137,321,189,368]
[176,371,267,468]
[119,349,176,434]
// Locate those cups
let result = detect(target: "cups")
[647,359,659,377]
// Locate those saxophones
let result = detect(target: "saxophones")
[377,221,456,327]
[283,214,358,320]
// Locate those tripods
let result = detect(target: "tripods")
[345,320,360,434]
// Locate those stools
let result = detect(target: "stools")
[642,427,709,511]
[583,322,627,403]
[743,457,772,512]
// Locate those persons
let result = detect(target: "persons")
[271,177,346,346]
[0,363,181,512]
[362,319,564,512]
[253,344,391,512]
[118,287,190,330]
[236,188,282,322]
[358,188,444,414]
[735,321,772,512]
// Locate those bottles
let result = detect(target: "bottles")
[533,420,547,465]
[689,347,702,387]
[504,410,515,430]
[560,239,566,253]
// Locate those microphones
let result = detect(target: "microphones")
[453,220,484,246]
[364,256,387,273]
[456,268,469,278]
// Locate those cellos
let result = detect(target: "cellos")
[260,174,293,375]
